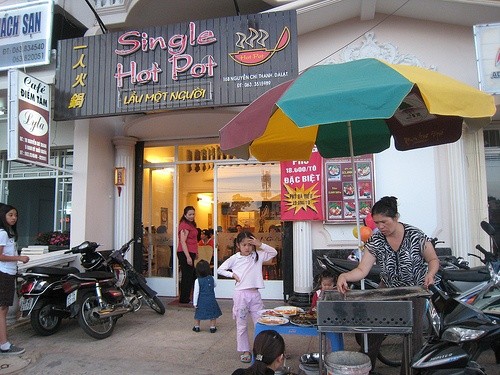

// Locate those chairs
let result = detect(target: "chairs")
[157,245,172,275]
[194,246,214,269]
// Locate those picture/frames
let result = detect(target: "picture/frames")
[114,167,125,185]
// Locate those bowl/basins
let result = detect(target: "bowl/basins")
[329,166,340,176]
[299,352,325,370]
[344,191,354,196]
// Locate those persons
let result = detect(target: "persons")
[231,330,287,375]
[0,205,30,355]
[192,259,223,333]
[310,270,336,309]
[144,206,283,304]
[336,196,440,375]
[217,231,278,363]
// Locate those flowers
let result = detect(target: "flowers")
[37,231,70,245]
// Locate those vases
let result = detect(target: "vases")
[49,245,70,252]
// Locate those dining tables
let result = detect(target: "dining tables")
[252,323,344,365]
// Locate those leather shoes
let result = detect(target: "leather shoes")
[210,327,216,332]
[192,326,200,332]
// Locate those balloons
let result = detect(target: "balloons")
[353,226,358,240]
[359,225,372,243]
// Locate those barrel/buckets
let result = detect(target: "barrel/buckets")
[324,351,372,375]
[298,364,326,375]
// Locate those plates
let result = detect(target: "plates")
[290,320,317,327]
[257,316,289,325]
[257,309,282,316]
[273,306,304,318]
[358,167,370,176]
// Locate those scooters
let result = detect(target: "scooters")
[15,241,114,337]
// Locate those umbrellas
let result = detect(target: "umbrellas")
[219,58,497,352]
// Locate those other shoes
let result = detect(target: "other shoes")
[241,354,251,363]
[0,341,26,354]
[180,298,190,303]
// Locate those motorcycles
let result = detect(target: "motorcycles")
[60,235,166,340]
[316,221,500,374]
